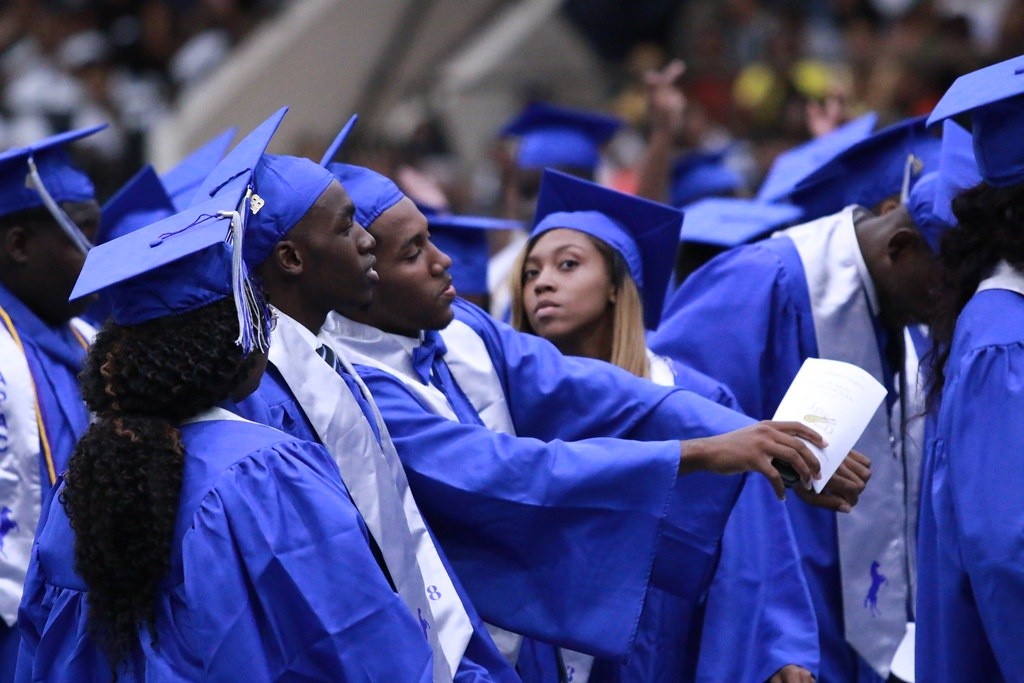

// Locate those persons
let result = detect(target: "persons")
[0,0,1024,683]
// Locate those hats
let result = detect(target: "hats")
[424,214,523,301]
[0,103,406,357]
[926,53,1024,188]
[662,110,983,312]
[496,101,623,173]
[523,166,685,333]
